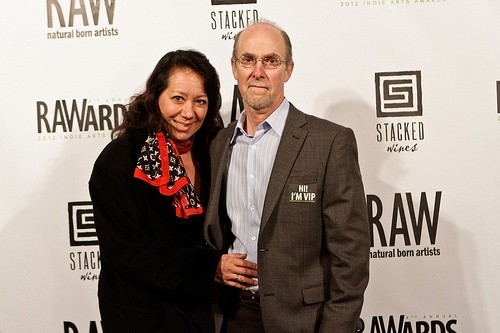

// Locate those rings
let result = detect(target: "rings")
[236,274,239,282]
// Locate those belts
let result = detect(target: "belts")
[231,290,261,306]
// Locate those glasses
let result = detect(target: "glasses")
[235,54,289,69]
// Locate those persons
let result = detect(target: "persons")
[202,22,370,333]
[88,49,259,333]
